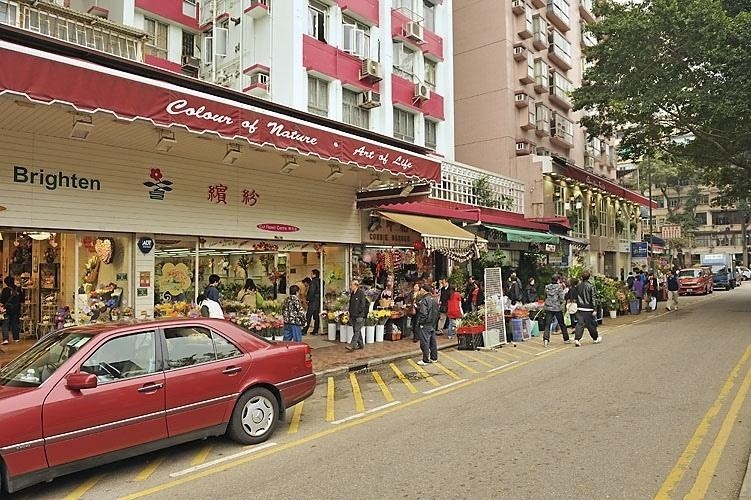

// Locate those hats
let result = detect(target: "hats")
[422,286,429,292]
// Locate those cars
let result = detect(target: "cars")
[736,264,751,288]
[0,315,318,500]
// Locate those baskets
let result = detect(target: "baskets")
[379,290,394,307]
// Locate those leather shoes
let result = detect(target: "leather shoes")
[310,332,317,335]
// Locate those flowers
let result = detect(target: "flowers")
[327,309,391,326]
[63,238,285,335]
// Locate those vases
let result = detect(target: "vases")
[328,323,386,345]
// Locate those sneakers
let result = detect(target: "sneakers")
[417,358,437,365]
[593,336,602,343]
[666,307,677,311]
[545,339,548,348]
[1,340,9,345]
[345,345,363,351]
[565,340,581,346]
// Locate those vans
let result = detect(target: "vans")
[679,266,714,296]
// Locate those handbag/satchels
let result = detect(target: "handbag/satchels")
[256,291,263,308]
[566,303,577,315]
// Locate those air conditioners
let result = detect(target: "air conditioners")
[362,21,432,101]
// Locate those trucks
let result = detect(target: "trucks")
[697,253,737,292]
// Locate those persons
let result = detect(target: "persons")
[296,277,312,307]
[418,285,439,366]
[345,280,366,351]
[621,267,680,311]
[302,269,326,336]
[197,295,226,319]
[205,274,221,301]
[283,285,306,341]
[1,276,26,345]
[238,279,258,311]
[411,271,603,348]
